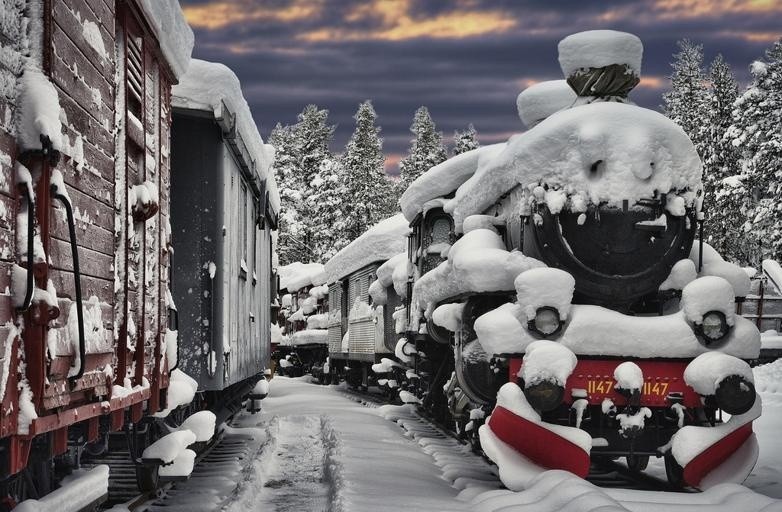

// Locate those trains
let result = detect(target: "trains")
[0,0,279,512]
[272,29,766,496]
